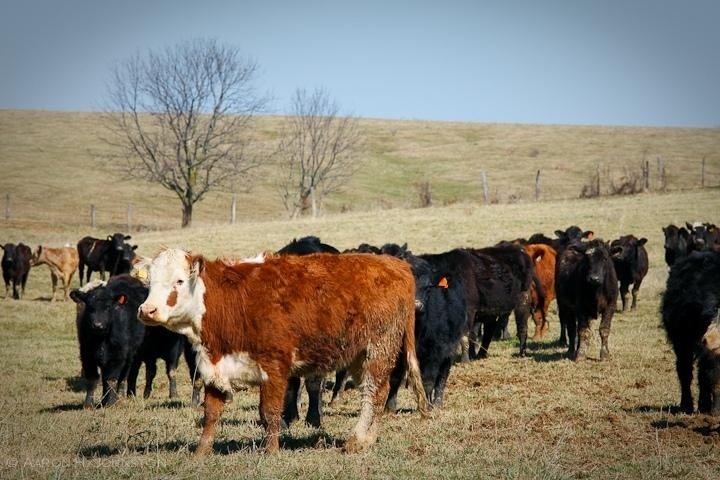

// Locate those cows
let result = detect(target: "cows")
[0,243,32,300]
[70,226,648,459]
[659,221,720,414]
[29,244,80,302]
